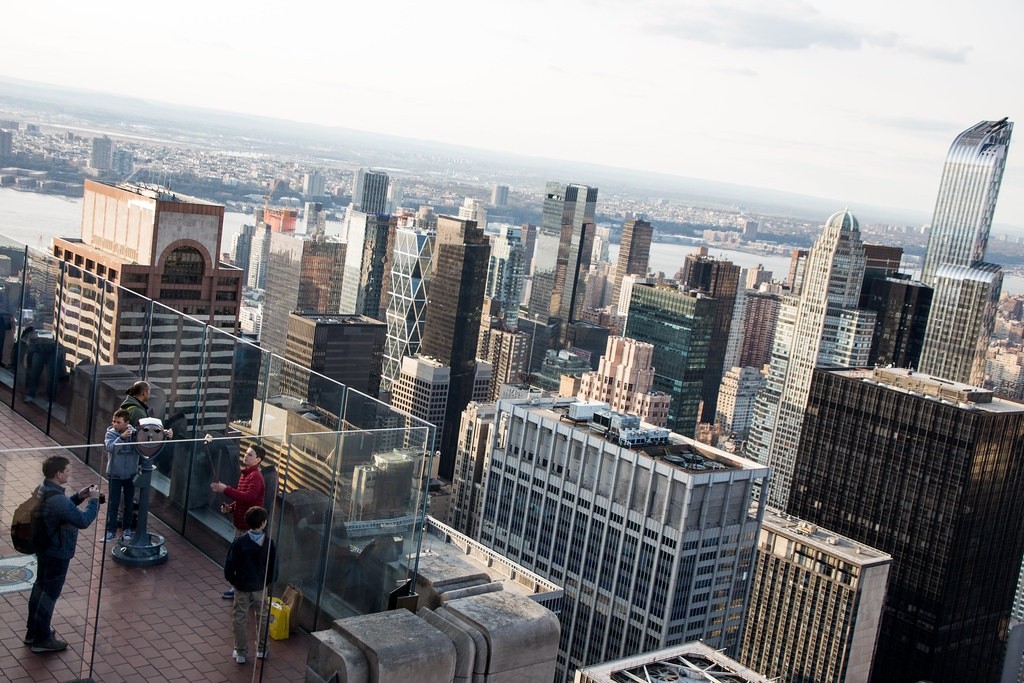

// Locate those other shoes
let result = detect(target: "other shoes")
[122,529,132,541]
[30,638,69,653]
[23,629,57,646]
[100,531,118,544]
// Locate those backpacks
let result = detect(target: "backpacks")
[11,484,59,556]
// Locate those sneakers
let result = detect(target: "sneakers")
[221,588,236,599]
[233,649,246,664]
[256,650,270,659]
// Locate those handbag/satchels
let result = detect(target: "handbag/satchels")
[281,583,303,634]
[266,596,290,640]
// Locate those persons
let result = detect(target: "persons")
[118,381,150,528]
[224,507,275,662]
[24,293,59,411]
[0,288,12,367]
[211,444,267,598]
[23,456,100,652]
[100,410,173,543]
